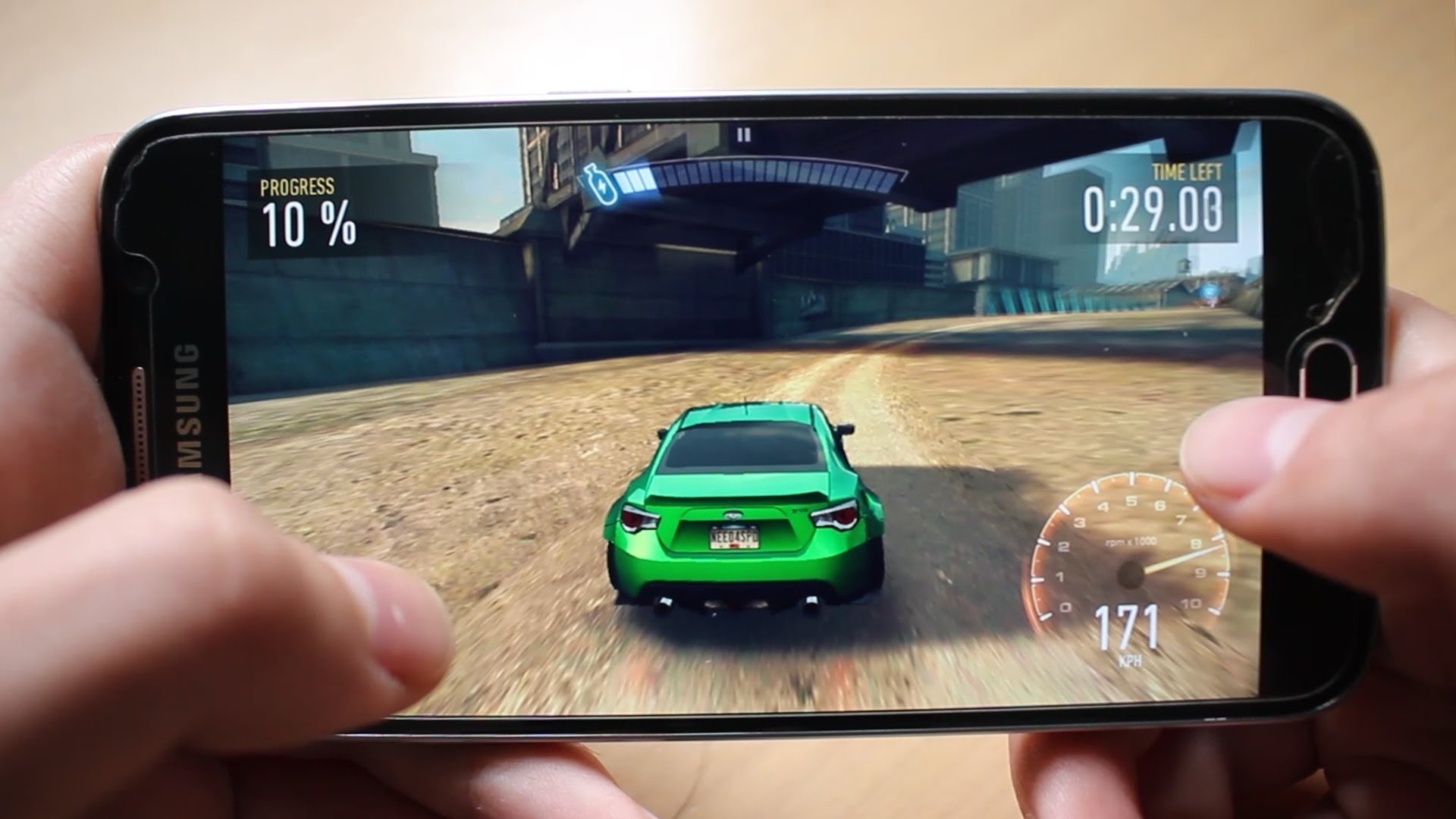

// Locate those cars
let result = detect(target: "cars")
[604,403,886,623]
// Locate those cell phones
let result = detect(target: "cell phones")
[95,88,1385,740]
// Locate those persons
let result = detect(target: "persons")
[0,134,1456,819]
